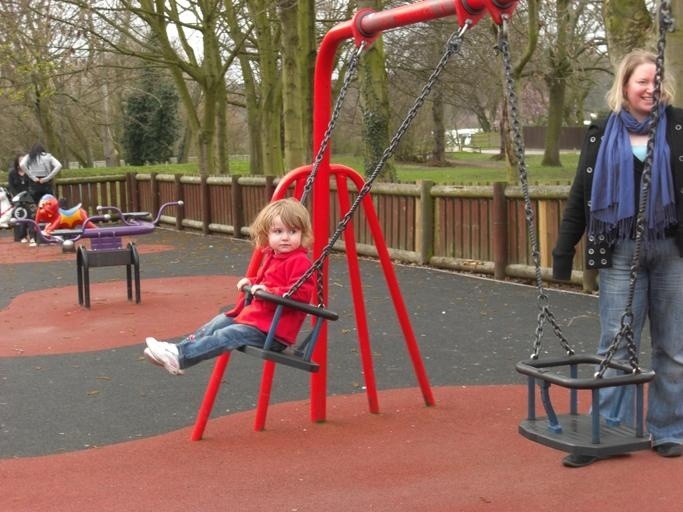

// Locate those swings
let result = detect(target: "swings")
[496,3,676,458]
[220,22,468,373]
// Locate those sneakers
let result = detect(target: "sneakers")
[654,443,683,457]
[562,453,597,468]
[21,237,35,243]
[144,336,184,376]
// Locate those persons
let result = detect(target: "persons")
[144,196,316,379]
[6,153,32,244]
[549,46,682,470]
[18,141,62,247]
